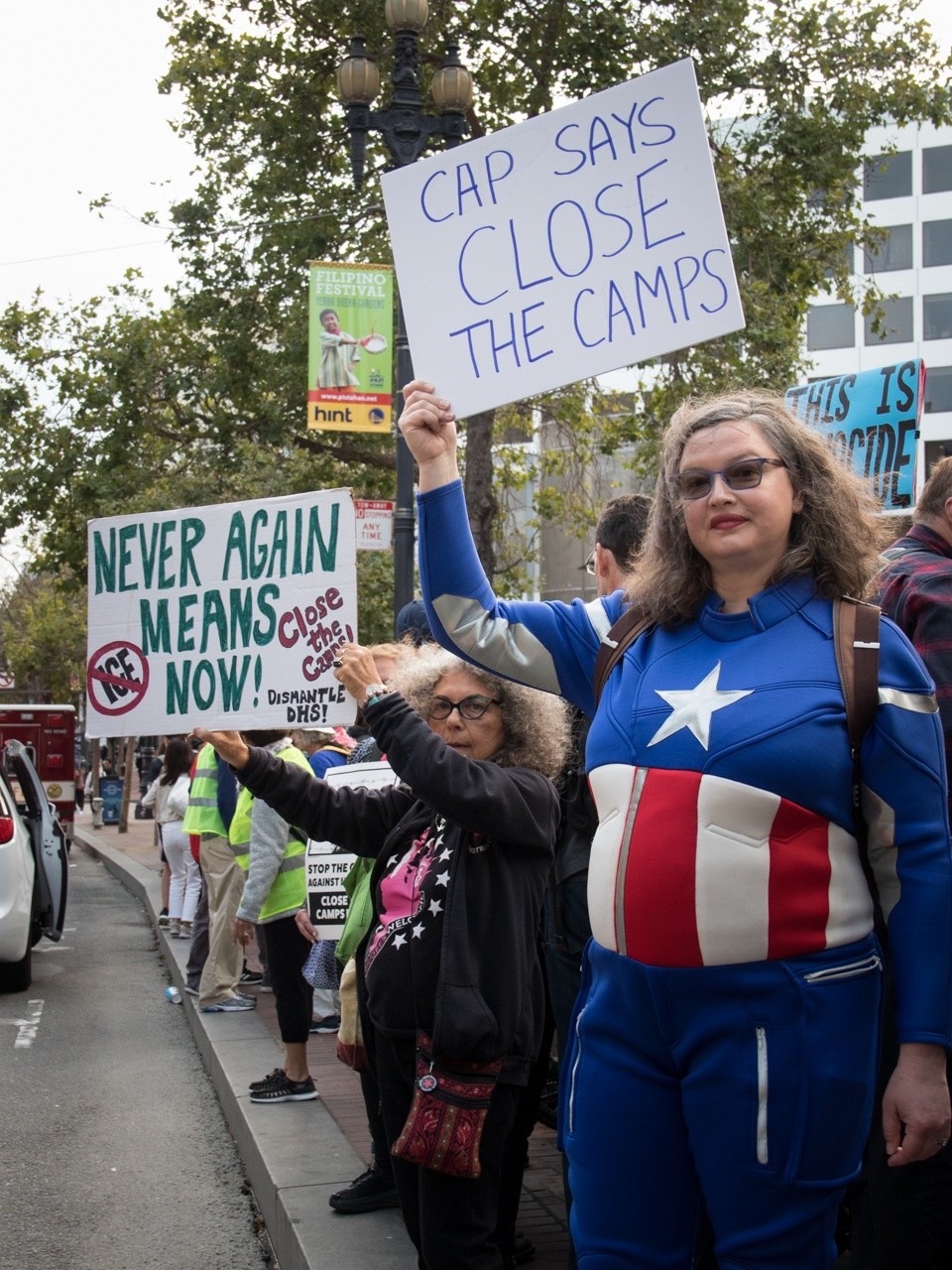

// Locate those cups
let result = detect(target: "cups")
[166,986,182,1004]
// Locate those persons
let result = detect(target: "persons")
[318,308,373,390]
[75,380,952,1270]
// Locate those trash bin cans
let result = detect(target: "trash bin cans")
[100,779,124,826]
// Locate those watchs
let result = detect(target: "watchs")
[365,683,388,694]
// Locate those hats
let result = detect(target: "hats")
[397,599,436,645]
[302,727,337,734]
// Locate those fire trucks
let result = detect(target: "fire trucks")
[1,702,77,857]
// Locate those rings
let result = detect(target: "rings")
[936,1137,948,1146]
[341,648,348,655]
[332,656,344,668]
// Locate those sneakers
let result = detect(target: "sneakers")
[248,1068,320,1103]
[201,988,257,1012]
[329,1162,400,1215]
[237,959,263,985]
[309,1015,340,1034]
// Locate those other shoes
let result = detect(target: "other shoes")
[178,924,192,938]
[513,1227,537,1266]
[535,1063,561,1129]
[184,972,202,995]
[259,985,273,993]
[158,907,170,929]
[170,922,181,937]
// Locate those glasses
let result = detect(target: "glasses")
[429,694,504,722]
[673,457,784,500]
[583,548,596,576]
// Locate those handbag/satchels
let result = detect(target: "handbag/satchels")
[301,940,344,989]
[391,1026,506,1178]
[335,957,373,1069]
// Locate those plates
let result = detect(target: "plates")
[364,334,387,353]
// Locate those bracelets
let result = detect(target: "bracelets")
[358,687,397,709]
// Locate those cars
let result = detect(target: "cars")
[0,737,68,993]
